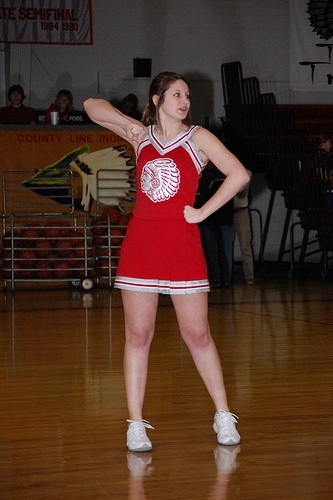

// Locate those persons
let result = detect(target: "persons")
[46,90,73,123]
[192,156,255,285]
[298,134,333,214]
[0,85,37,123]
[84,71,251,450]
[116,94,141,120]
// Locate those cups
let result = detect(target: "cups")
[50,112,59,125]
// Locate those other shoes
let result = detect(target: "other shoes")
[210,283,221,287]
[222,282,230,286]
[248,280,255,284]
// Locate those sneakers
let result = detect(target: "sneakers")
[126,419,155,451]
[213,411,241,445]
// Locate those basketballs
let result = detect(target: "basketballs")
[0,205,133,278]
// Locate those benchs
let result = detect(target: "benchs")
[222,104,333,234]
